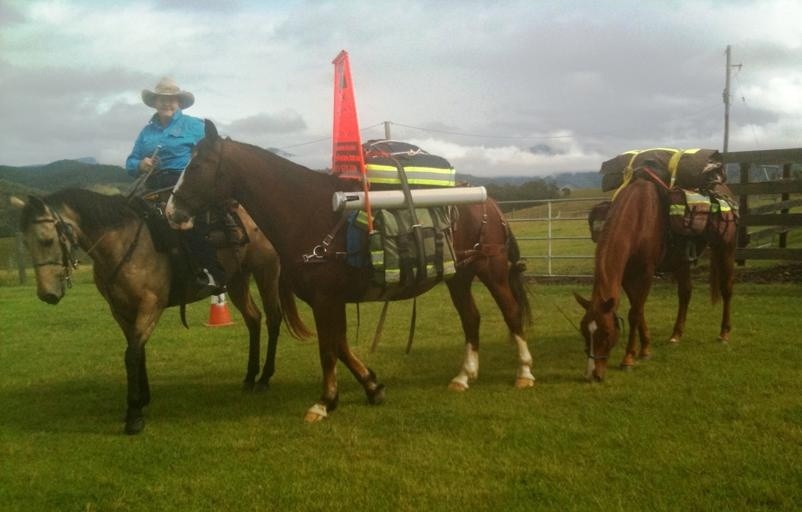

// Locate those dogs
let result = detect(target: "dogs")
[7,184,315,435]
[165,117,537,425]
[572,174,742,384]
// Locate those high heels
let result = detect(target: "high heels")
[206,294,233,326]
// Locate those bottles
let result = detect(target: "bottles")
[139,75,196,111]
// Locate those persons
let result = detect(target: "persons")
[125,75,229,296]
